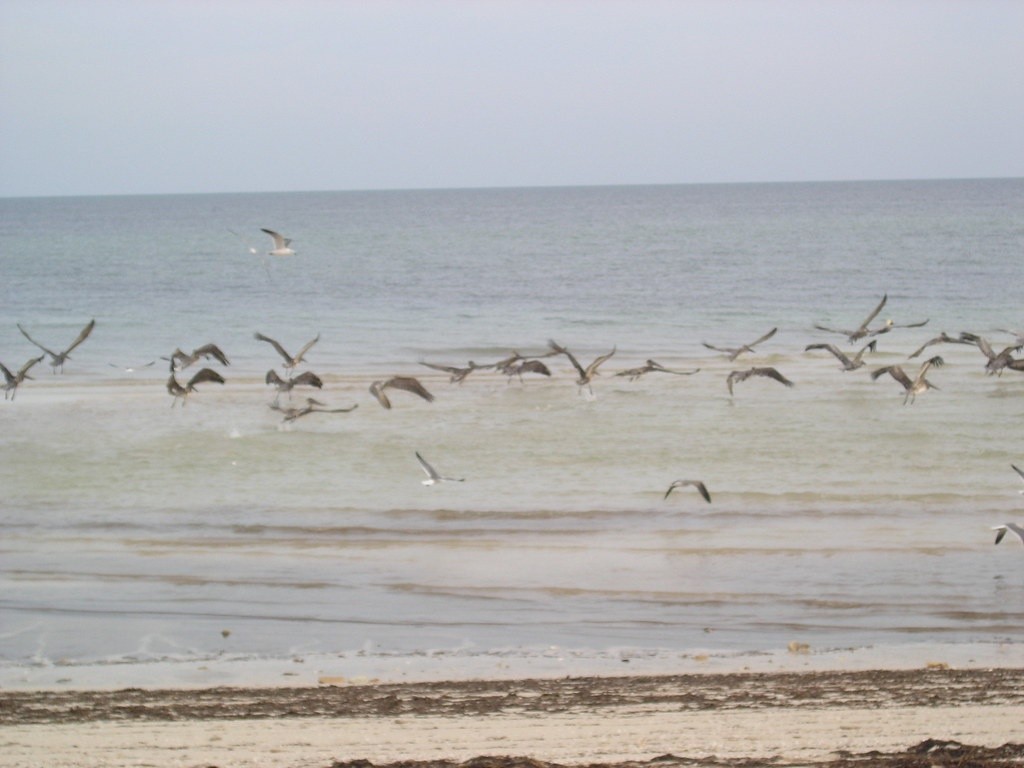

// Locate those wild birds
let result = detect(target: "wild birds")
[108,344,230,407]
[704,327,795,397]
[0,319,94,402]
[993,463,1024,546]
[369,374,436,409]
[261,228,295,256]
[415,451,466,488]
[416,341,615,395]
[663,479,711,503]
[617,359,700,383]
[254,331,358,431]
[804,293,1024,406]
